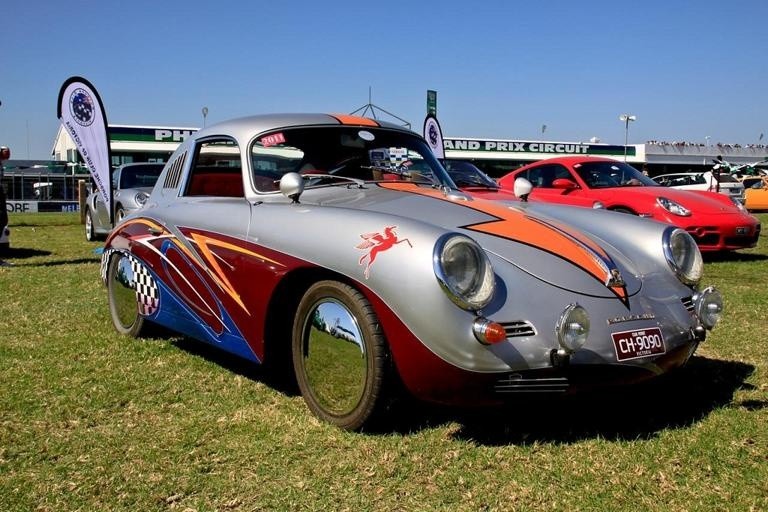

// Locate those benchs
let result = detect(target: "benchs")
[190,172,280,198]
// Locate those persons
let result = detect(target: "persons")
[646,140,768,149]
